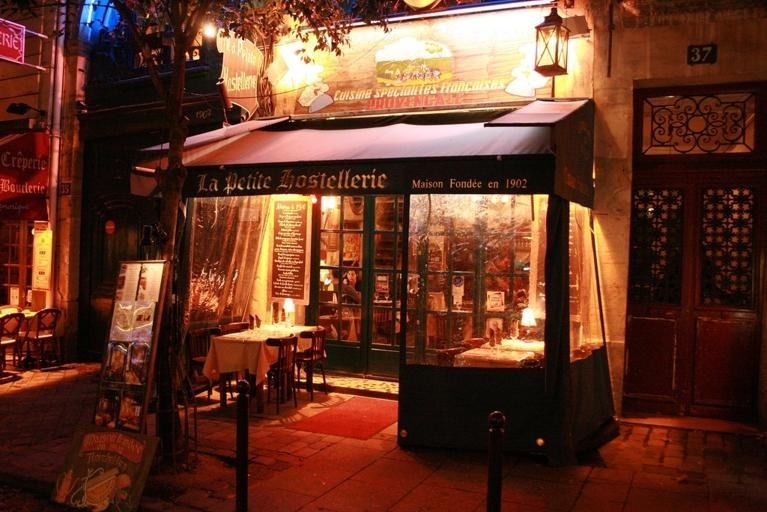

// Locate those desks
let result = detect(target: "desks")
[319,313,361,340]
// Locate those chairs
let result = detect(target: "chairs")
[0,305,62,372]
[187,322,328,415]
[435,315,456,349]
[374,303,392,344]
[437,337,591,368]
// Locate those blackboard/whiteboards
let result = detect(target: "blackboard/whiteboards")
[268,196,313,306]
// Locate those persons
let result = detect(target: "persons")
[345,269,361,301]
[329,265,361,303]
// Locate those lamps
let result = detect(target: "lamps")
[7,102,45,117]
[520,308,537,342]
[283,298,295,327]
[535,7,570,97]
